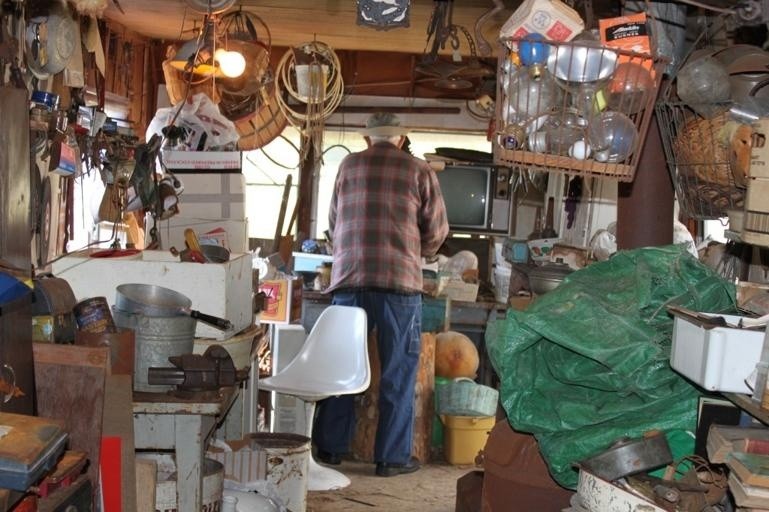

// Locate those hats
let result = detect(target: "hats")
[358,113,409,138]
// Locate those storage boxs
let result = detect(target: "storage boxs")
[740,116,769,250]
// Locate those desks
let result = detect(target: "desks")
[301,278,512,387]
[132,380,246,512]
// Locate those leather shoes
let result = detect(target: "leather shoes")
[376,455,421,477]
[317,449,342,465]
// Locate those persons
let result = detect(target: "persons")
[311,108,450,478]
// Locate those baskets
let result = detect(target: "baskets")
[492,34,673,183]
[654,98,751,222]
[432,377,497,418]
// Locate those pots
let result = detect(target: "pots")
[116,282,235,335]
[503,256,576,295]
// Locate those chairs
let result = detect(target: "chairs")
[258,304,372,491]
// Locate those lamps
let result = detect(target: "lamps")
[182,1,246,85]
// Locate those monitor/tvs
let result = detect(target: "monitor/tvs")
[430,164,513,232]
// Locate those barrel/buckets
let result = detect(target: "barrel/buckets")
[438,413,496,465]
[217,63,289,152]
[111,304,197,394]
[244,431,312,512]
[161,57,222,106]
[135,451,225,512]
[294,63,330,99]
[428,376,452,447]
[349,330,437,467]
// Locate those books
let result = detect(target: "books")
[704,422,769,512]
